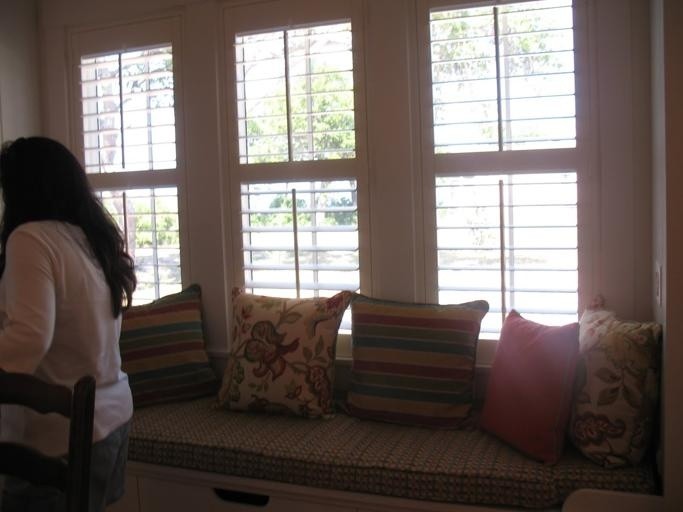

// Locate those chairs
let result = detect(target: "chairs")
[1,368,96,512]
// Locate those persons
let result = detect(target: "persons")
[1,130,138,512]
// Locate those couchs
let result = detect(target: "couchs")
[100,358,662,512]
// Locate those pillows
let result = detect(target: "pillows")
[343,291,489,431]
[119,285,220,408]
[211,288,352,421]
[562,295,663,473]
[478,309,581,464]
